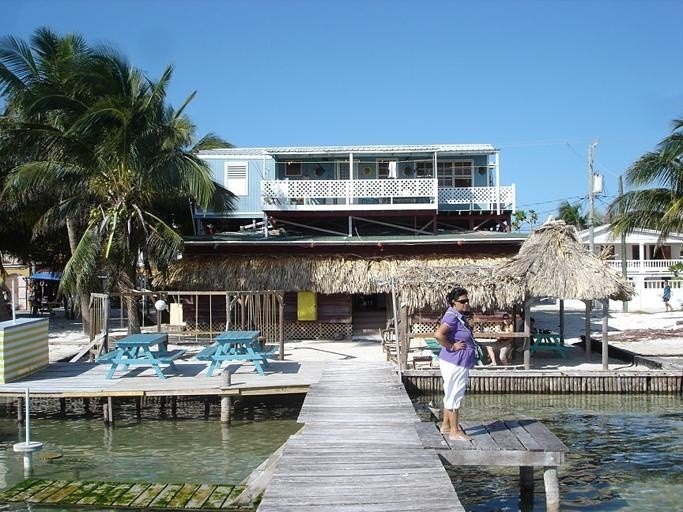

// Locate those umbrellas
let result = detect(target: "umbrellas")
[24,265,63,282]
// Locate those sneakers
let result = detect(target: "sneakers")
[441,428,471,441]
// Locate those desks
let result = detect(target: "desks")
[206,331,270,376]
[106,333,178,380]
[530,330,567,359]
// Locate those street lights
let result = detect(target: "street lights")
[583,141,600,363]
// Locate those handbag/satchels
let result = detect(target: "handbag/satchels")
[474,340,482,360]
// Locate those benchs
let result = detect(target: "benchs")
[95,345,278,380]
[424,338,442,357]
[530,342,576,359]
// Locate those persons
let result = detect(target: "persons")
[661,279,674,312]
[485,313,514,366]
[514,311,538,357]
[26,281,56,316]
[432,286,476,441]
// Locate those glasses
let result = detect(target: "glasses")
[456,299,469,304]
